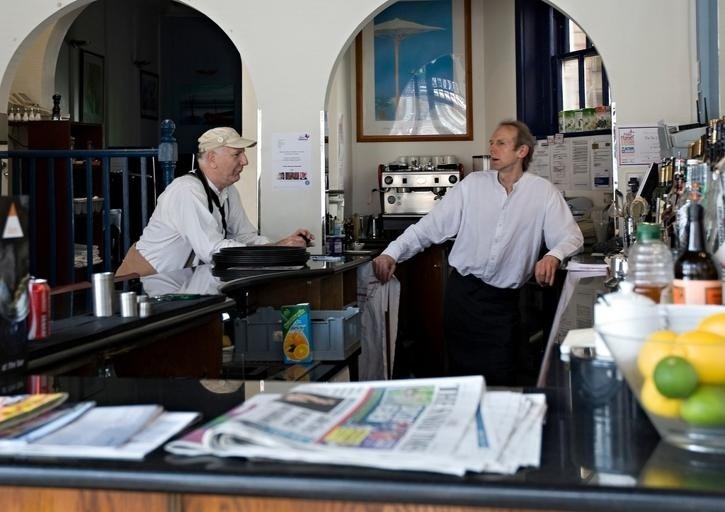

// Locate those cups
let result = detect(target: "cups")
[591,282,658,365]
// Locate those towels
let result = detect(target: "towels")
[74,244,104,268]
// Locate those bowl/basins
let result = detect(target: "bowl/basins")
[593,305,719,456]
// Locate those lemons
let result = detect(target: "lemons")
[676,382,725,430]
[653,355,699,400]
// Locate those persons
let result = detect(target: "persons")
[114,127,315,279]
[137,264,230,301]
[372,121,585,386]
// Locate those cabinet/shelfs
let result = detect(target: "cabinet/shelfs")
[8,121,109,288]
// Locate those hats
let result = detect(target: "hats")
[197,126,258,152]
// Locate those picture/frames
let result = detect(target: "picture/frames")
[79,48,106,122]
[355,0,474,143]
[139,69,160,121]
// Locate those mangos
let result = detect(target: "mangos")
[698,311,725,339]
[640,376,680,418]
[637,329,678,378]
[673,330,725,384]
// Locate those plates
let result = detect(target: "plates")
[346,251,373,254]
[210,243,311,271]
[313,256,348,262]
[397,154,458,171]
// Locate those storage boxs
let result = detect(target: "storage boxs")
[235,306,363,360]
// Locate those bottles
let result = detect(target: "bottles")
[702,115,725,167]
[92,273,114,318]
[25,278,51,339]
[121,291,137,317]
[139,302,151,317]
[687,159,698,180]
[627,218,634,246]
[627,224,673,282]
[675,203,719,281]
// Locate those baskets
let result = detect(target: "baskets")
[74,198,106,215]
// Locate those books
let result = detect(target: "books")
[0,392,70,431]
